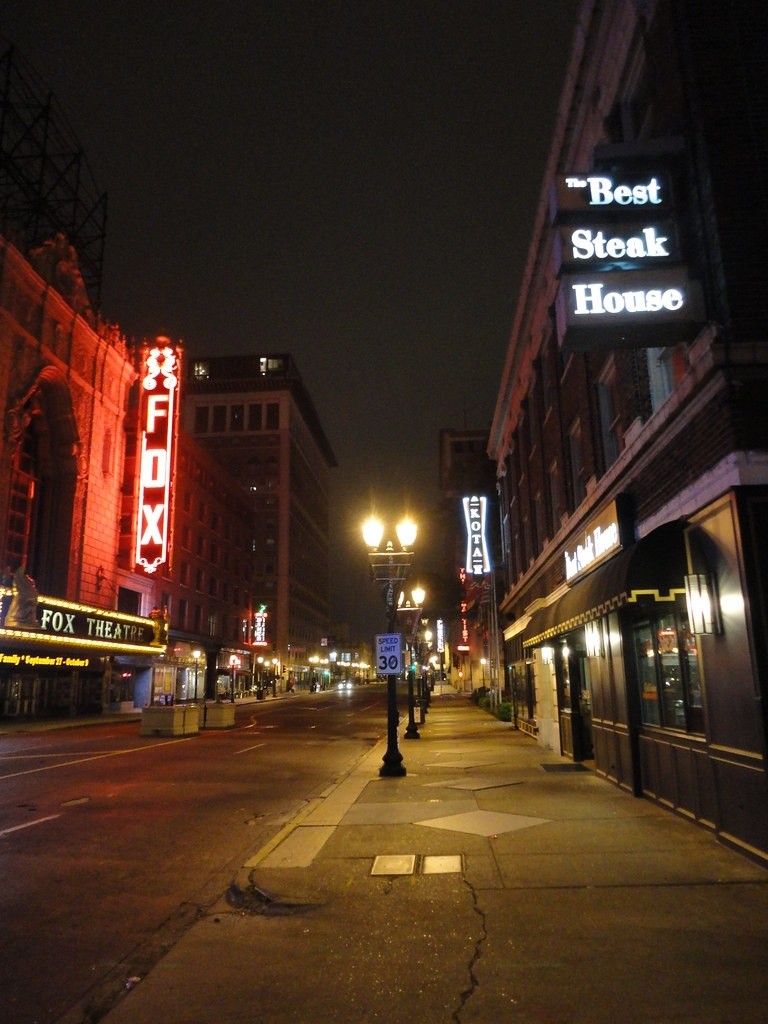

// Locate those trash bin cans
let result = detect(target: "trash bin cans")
[413,699,425,724]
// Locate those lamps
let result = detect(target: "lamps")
[683,572,722,636]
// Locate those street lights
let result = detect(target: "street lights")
[193,650,201,702]
[229,655,238,702]
[358,509,433,778]
[256,658,278,700]
